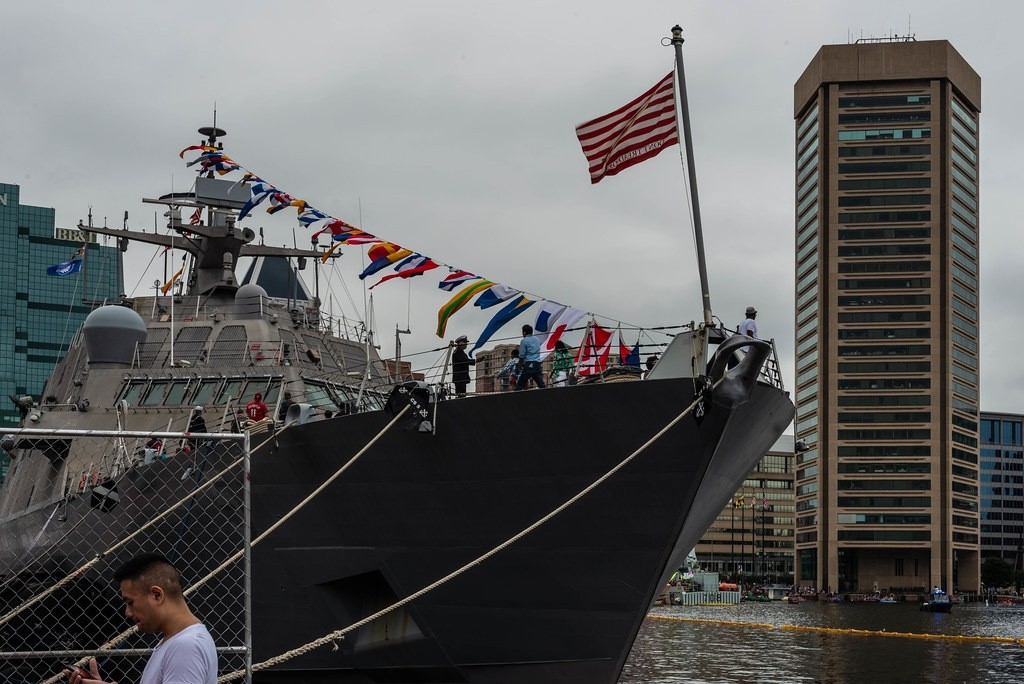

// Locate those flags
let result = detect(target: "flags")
[161,146,446,297]
[619,322,641,380]
[436,268,589,362]
[47,248,85,277]
[569,319,615,376]
[733,494,768,509]
[575,58,680,185]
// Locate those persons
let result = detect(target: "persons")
[187,406,207,451]
[246,393,270,422]
[739,307,758,355]
[789,584,815,594]
[452,335,485,398]
[497,349,530,391]
[147,437,162,452]
[644,356,658,380]
[279,391,296,421]
[933,585,938,593]
[515,325,545,390]
[549,340,574,387]
[176,439,189,454]
[63,553,217,684]
[231,410,249,434]
[325,403,349,418]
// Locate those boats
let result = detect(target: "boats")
[919,584,955,613]
[0,98,798,684]
[742,584,899,605]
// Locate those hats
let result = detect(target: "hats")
[745,307,757,315]
[285,391,291,398]
[195,406,203,410]
[255,393,261,400]
[455,335,469,342]
